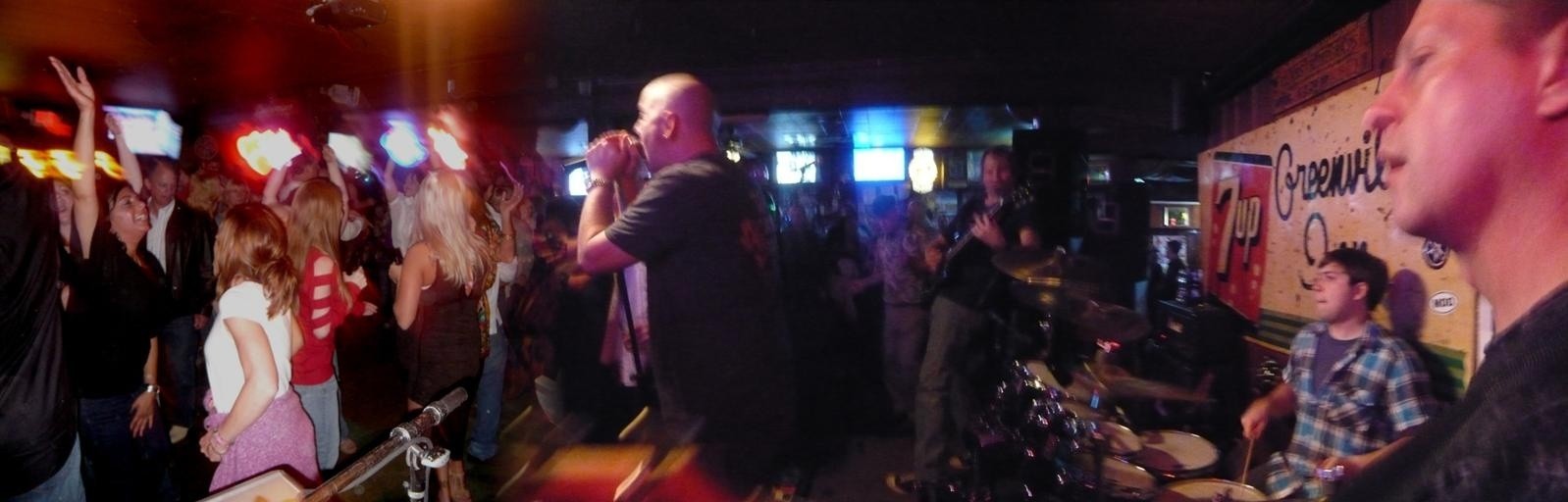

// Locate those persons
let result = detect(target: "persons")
[575,71,1192,500]
[1239,249,1440,502]
[2,54,640,502]
[1321,0,1567,501]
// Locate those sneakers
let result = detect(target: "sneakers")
[885,473,932,496]
